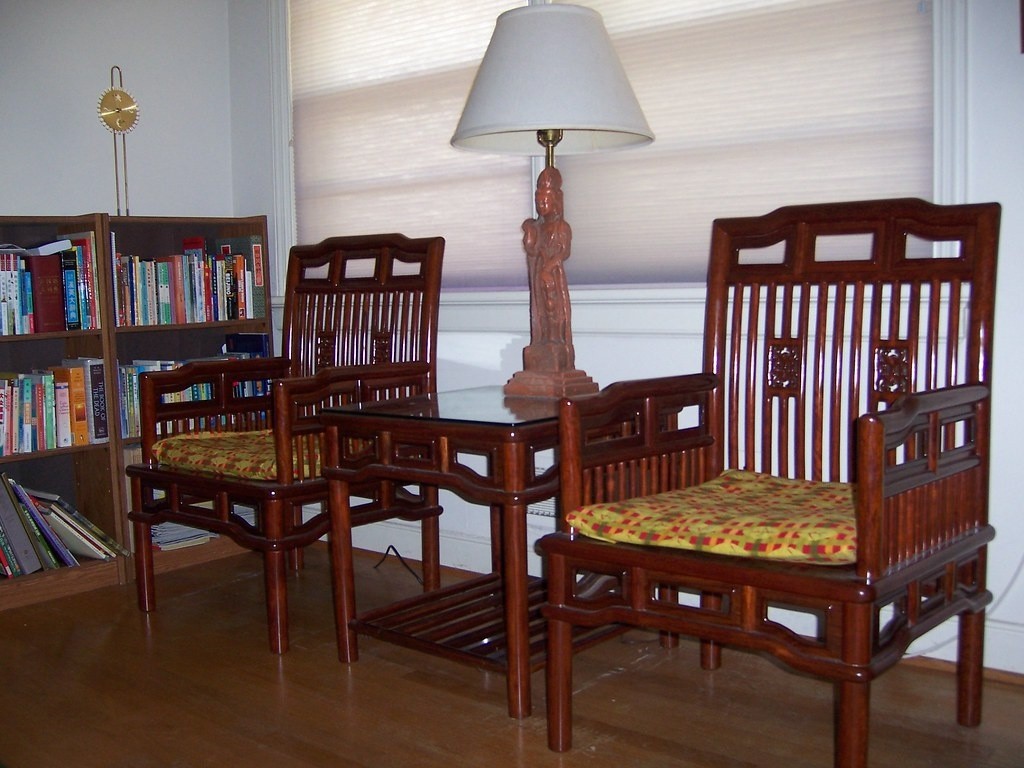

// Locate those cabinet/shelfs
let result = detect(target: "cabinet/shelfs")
[0,213,274,610]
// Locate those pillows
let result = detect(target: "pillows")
[150,426,376,483]
[561,466,860,564]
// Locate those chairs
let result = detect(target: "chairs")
[125,233,445,655]
[532,197,1003,768]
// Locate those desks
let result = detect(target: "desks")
[316,386,684,720]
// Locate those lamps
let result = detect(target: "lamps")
[450,3,654,400]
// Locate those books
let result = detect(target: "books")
[0,229,103,336]
[0,356,108,457]
[114,332,270,440]
[109,231,268,327]
[151,489,255,552]
[0,472,131,578]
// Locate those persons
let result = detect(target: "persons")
[520,166,575,372]
